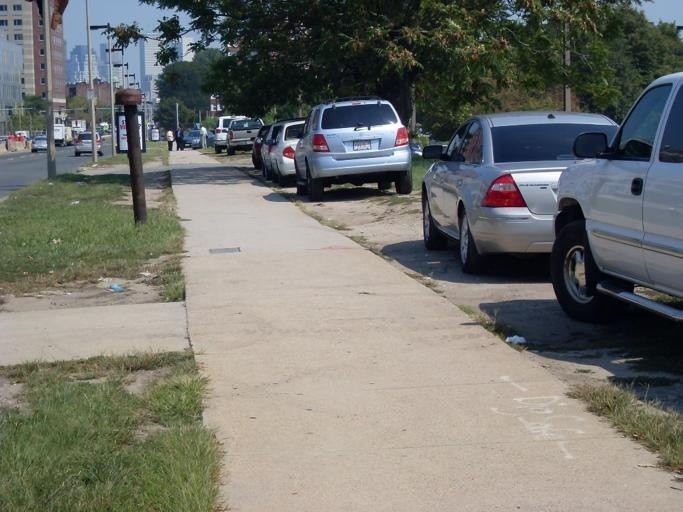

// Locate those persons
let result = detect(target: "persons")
[7,133,15,141]
[174,127,183,151]
[18,133,24,142]
[199,124,207,150]
[165,128,175,151]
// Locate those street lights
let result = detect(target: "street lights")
[103,42,152,123]
[87,22,115,154]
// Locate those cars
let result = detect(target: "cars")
[418,112,620,276]
[0,121,158,156]
[180,96,413,201]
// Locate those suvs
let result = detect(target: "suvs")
[550,68,681,341]
